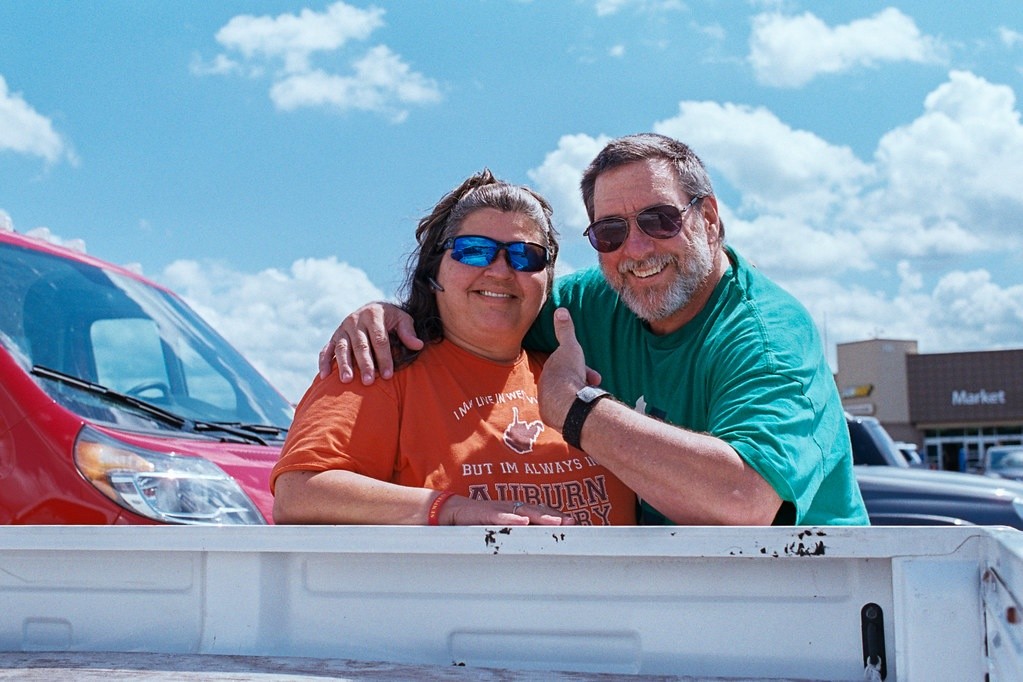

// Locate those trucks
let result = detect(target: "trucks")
[2,523,1023,681]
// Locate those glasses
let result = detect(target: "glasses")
[582,194,698,254]
[435,235,553,272]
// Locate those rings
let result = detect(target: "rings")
[513,502,524,514]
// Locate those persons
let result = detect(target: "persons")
[319,133,874,526]
[270,168,637,526]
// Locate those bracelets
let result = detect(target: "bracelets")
[561,384,612,452]
[428,491,455,526]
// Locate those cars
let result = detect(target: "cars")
[972,446,1023,484]
[0,228,300,527]
[844,410,909,469]
[853,464,1023,532]
[894,443,926,470]
